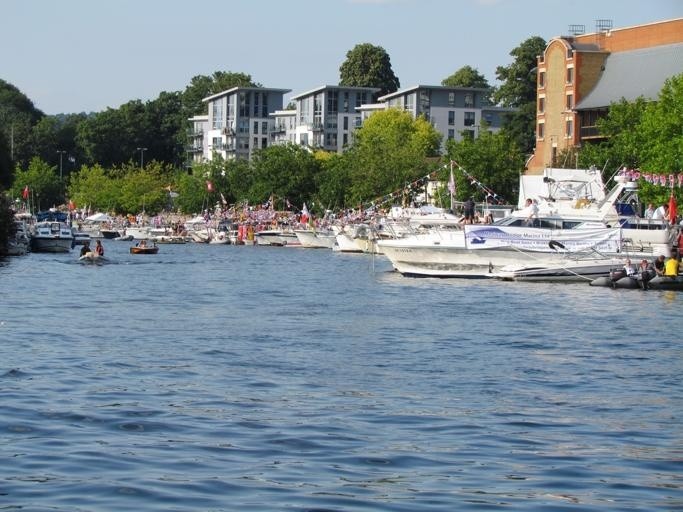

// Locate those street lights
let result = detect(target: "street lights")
[137,148,148,168]
[57,151,66,178]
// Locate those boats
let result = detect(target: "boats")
[100,227,238,244]
[9,211,92,254]
[251,175,683,289]
[130,241,159,253]
[79,252,110,265]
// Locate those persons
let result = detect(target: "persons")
[622,199,683,279]
[8,198,27,215]
[67,198,539,258]
